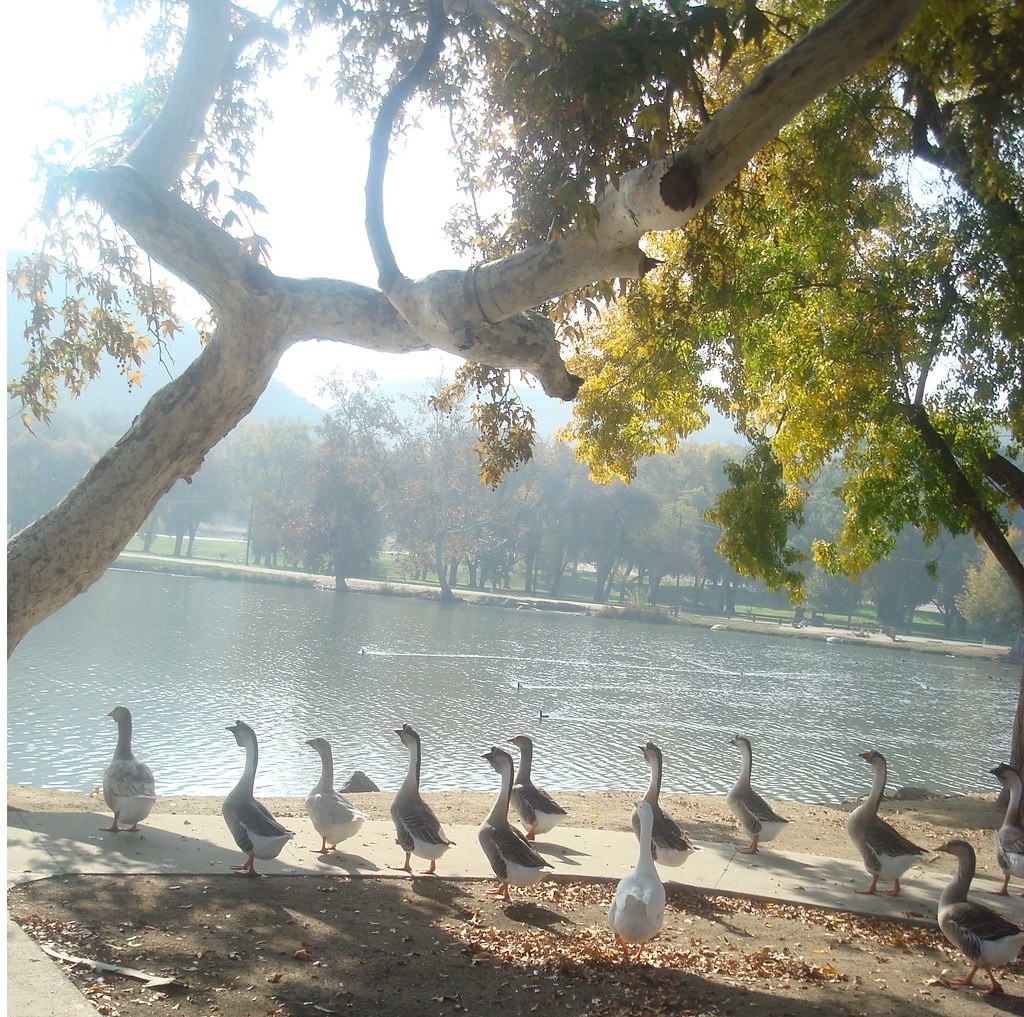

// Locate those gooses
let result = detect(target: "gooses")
[102,705,1024,995]
